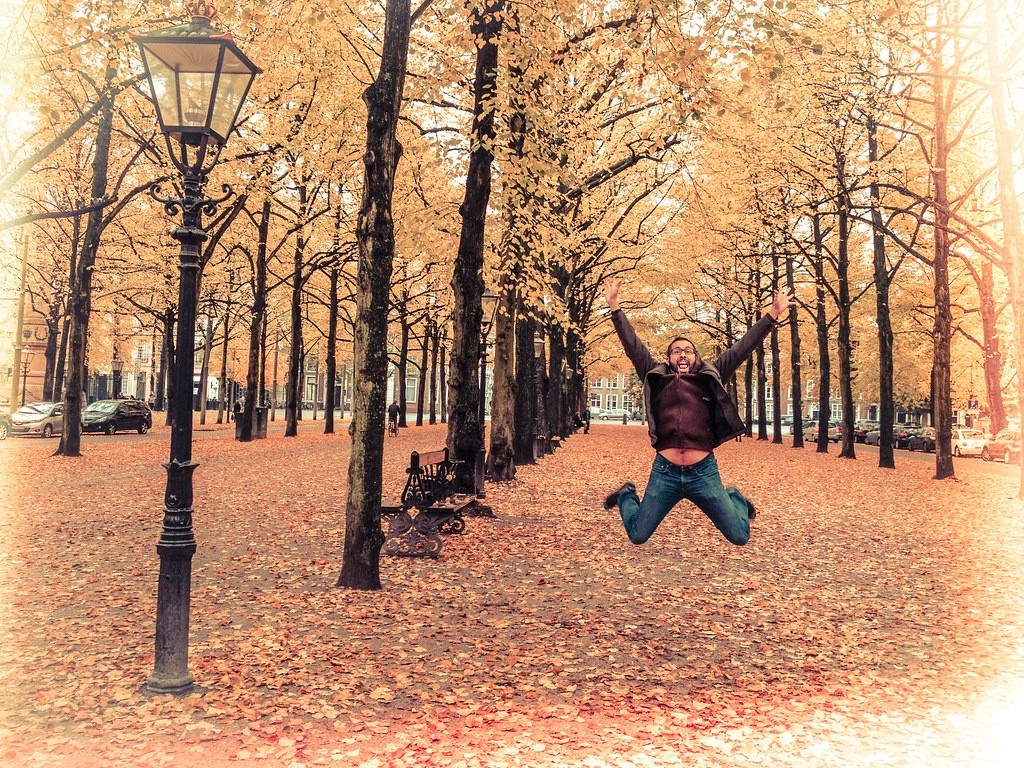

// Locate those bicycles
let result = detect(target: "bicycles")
[387,419,400,437]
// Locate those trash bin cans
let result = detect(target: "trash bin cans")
[235,412,258,440]
[256,407,268,439]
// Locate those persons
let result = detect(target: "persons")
[206,396,229,410]
[574,412,589,434]
[149,391,155,410]
[583,406,591,434]
[233,400,241,422]
[388,399,402,437]
[120,392,125,399]
[603,281,798,545]
[131,394,136,400]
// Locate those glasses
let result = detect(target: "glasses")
[669,348,695,356]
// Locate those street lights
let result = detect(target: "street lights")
[111,355,124,399]
[19,345,36,405]
[125,2,265,702]
[475,286,503,500]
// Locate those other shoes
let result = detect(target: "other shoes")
[727,487,756,519]
[603,482,635,510]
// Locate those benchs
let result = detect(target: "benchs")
[381,447,478,558]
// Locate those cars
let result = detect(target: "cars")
[864,427,880,446]
[951,429,993,458]
[903,422,922,429]
[82,398,153,435]
[979,428,1022,465]
[744,416,772,426]
[0,409,13,441]
[892,427,919,449]
[908,427,937,453]
[631,411,647,421]
[832,418,880,443]
[10,402,83,438]
[803,420,839,444]
[780,415,810,435]
[952,423,968,430]
[599,408,632,421]
